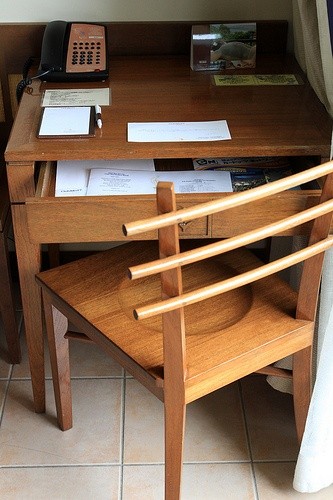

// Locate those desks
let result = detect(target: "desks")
[4,45,333,413]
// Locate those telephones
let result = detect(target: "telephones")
[16,20,110,104]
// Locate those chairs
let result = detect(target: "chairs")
[36,158,333,500]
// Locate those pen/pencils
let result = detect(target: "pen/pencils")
[94,103,105,131]
